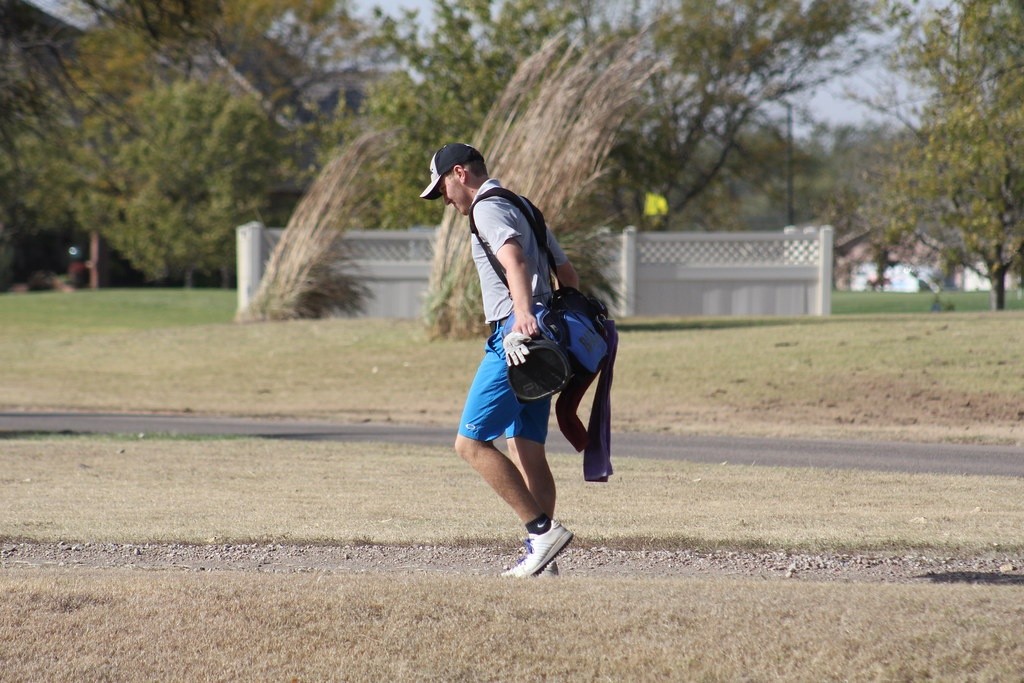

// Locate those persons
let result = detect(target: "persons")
[416,142,579,577]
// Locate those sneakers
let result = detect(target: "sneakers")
[500,518,575,579]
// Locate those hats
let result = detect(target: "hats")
[421,142,485,200]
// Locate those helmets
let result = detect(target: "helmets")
[506,339,573,401]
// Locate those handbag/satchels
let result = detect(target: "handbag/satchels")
[501,285,612,389]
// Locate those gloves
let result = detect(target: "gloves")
[502,332,531,367]
